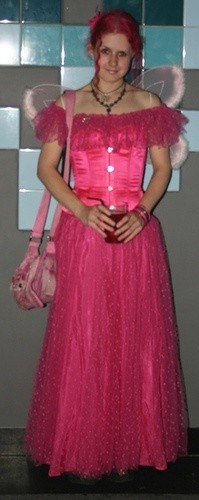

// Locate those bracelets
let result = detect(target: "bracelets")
[134,203,151,222]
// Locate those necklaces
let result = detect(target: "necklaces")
[89,78,128,112]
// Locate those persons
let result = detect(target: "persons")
[25,9,190,486]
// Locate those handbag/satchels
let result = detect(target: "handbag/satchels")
[11,253,55,310]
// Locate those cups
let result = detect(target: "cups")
[103,201,128,243]
